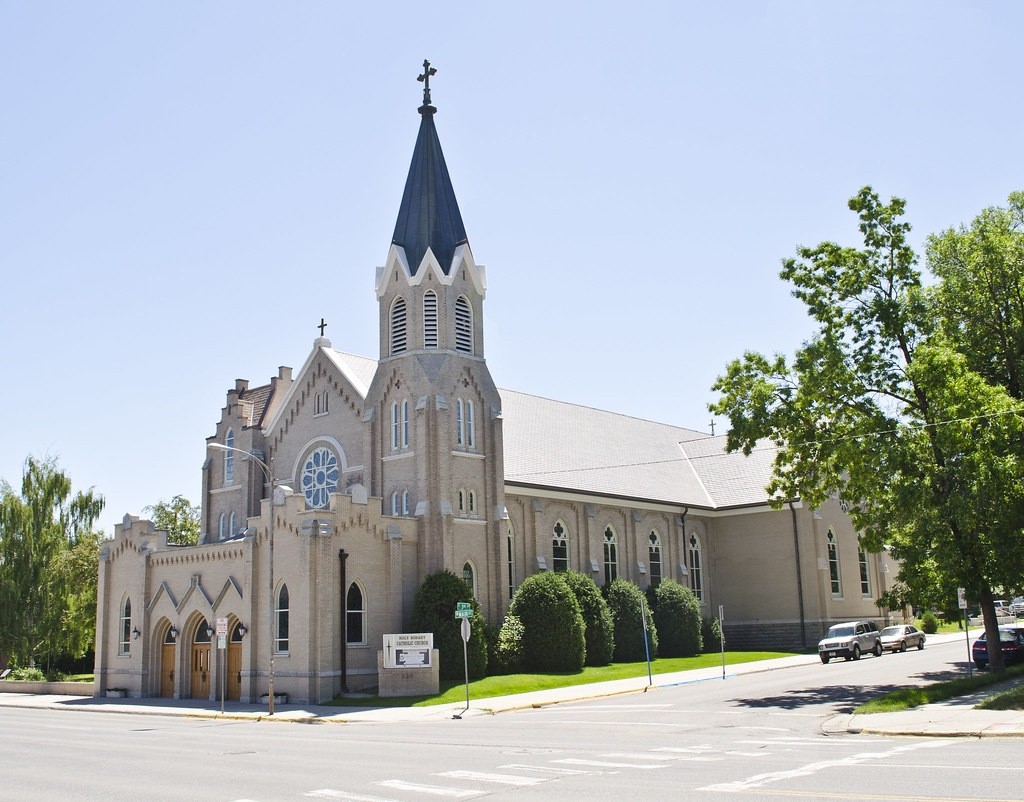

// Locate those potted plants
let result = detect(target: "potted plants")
[258,692,289,705]
[105,686,128,698]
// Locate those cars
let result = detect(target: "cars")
[992,600,1010,617]
[879,624,927,653]
[972,627,1024,668]
[1009,596,1024,616]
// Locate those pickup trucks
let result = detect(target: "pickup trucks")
[818,621,883,664]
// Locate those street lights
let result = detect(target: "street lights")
[207,442,275,714]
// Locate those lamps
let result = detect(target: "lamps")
[206,625,215,638]
[132,625,141,640]
[170,624,180,638]
[238,623,247,637]
